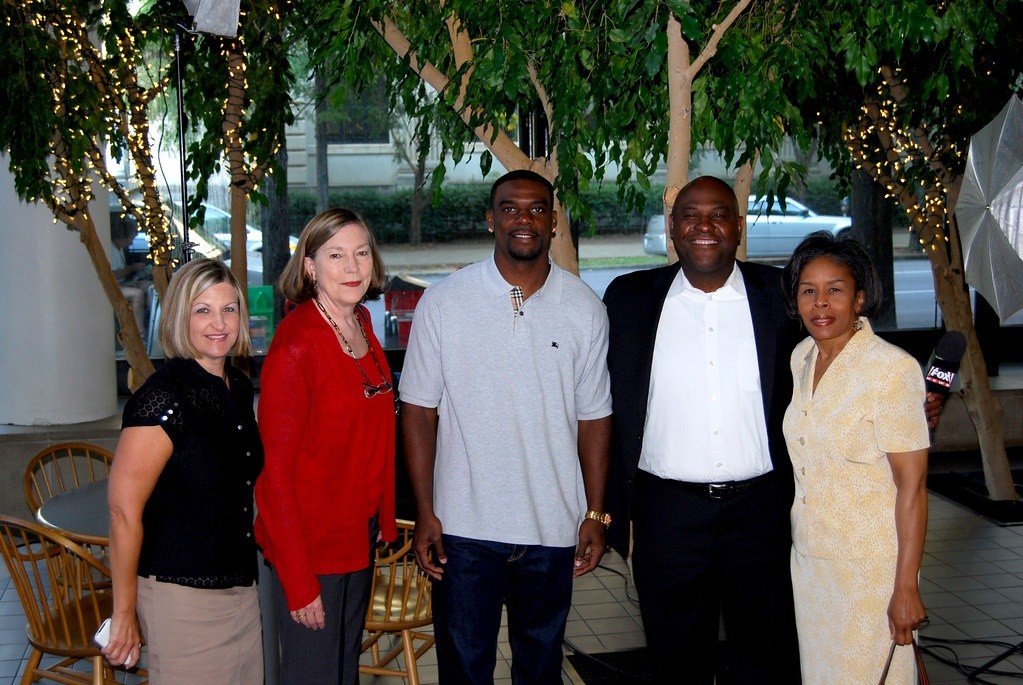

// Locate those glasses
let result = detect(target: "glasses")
[362,380,393,399]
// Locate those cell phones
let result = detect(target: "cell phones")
[95,618,141,665]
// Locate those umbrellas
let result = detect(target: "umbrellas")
[955,94,1022,326]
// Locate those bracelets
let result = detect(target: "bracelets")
[584,510,612,526]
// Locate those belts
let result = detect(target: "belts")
[639,471,775,500]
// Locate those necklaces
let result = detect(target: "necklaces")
[316,297,390,392]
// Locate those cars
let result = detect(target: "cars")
[173,200,299,256]
[114,197,267,286]
[643,193,853,264]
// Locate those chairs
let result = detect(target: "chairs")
[22,442,114,610]
[358,519,434,685]
[0,509,149,685]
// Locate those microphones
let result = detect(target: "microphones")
[924,330,967,396]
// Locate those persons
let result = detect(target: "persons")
[101,257,265,685]
[396,169,614,685]
[255,206,398,685]
[602,176,811,685]
[781,230,928,685]
[110,213,154,341]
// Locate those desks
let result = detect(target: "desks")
[149,286,409,567]
[36,478,112,592]
